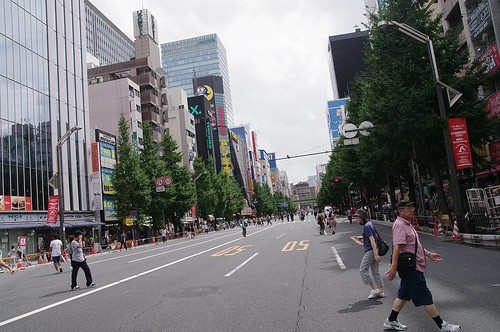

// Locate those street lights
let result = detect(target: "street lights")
[377,20,466,232]
[194,172,206,229]
[57,125,82,257]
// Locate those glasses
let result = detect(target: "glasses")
[406,207,415,210]
[355,216,359,217]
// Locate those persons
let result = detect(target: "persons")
[0,181,500,275]
[49,235,64,273]
[383,199,462,332]
[353,208,387,299]
[71,231,97,290]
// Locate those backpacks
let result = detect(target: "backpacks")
[318,216,322,220]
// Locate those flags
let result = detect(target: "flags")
[448,117,474,170]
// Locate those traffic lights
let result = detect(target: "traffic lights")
[331,177,340,183]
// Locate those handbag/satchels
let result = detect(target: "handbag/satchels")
[373,226,389,256]
[390,252,416,270]
[244,222,247,226]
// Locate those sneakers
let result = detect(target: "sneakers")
[89,282,97,288]
[72,286,80,290]
[383,318,407,330]
[437,320,462,332]
[59,267,63,273]
[368,289,381,299]
[377,293,386,298]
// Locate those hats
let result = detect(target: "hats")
[354,209,367,220]
[395,200,415,207]
[75,230,85,235]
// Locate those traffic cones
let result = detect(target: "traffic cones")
[17,258,22,268]
[451,218,462,242]
[436,219,445,235]
